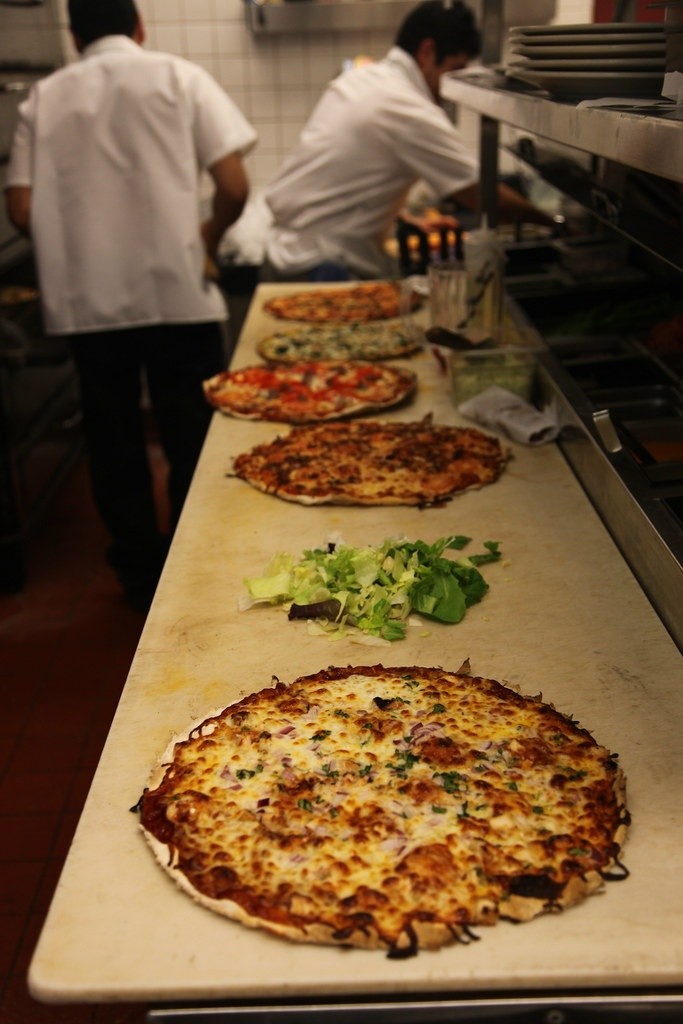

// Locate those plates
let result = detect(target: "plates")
[504,19,667,101]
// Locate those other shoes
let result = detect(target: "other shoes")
[127,576,159,610]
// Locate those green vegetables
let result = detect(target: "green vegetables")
[248,534,503,641]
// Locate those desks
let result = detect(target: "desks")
[29,283,683,1023]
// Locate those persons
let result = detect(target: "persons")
[3,0,256,611]
[265,1,568,283]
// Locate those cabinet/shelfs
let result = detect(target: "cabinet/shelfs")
[439,65,682,652]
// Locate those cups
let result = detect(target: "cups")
[428,261,500,344]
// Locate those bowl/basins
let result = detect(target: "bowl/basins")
[449,348,539,410]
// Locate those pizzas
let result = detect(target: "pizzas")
[140,663,627,947]
[204,361,415,422]
[233,412,505,504]
[257,322,424,361]
[263,282,420,321]
